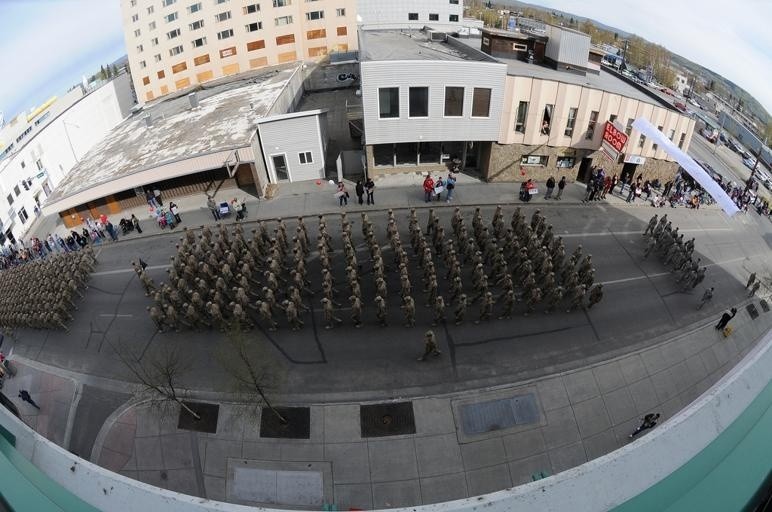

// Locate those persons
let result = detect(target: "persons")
[356,177,375,206]
[146,205,604,334]
[714,308,737,330]
[336,180,348,209]
[146,186,164,209]
[745,271,761,299]
[424,171,457,204]
[543,175,567,201]
[155,201,182,230]
[641,214,707,296]
[423,330,440,359]
[231,195,248,221]
[1,214,143,269]
[130,261,157,297]
[19,389,41,410]
[207,196,220,222]
[697,288,714,310]
[519,179,534,202]
[581,165,716,210]
[628,413,660,437]
[716,173,772,220]
[2,263,96,335]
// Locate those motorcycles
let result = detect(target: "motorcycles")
[119,217,134,235]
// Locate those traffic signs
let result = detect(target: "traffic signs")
[37,172,45,179]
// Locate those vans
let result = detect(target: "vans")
[681,156,722,192]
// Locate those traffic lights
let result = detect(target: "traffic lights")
[23,182,28,192]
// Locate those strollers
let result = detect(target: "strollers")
[219,201,232,220]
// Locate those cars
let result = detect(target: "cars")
[601,53,681,100]
[693,111,772,193]
[28,181,31,187]
[673,100,687,113]
[688,99,701,109]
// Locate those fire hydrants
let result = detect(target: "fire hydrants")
[724,323,734,339]
